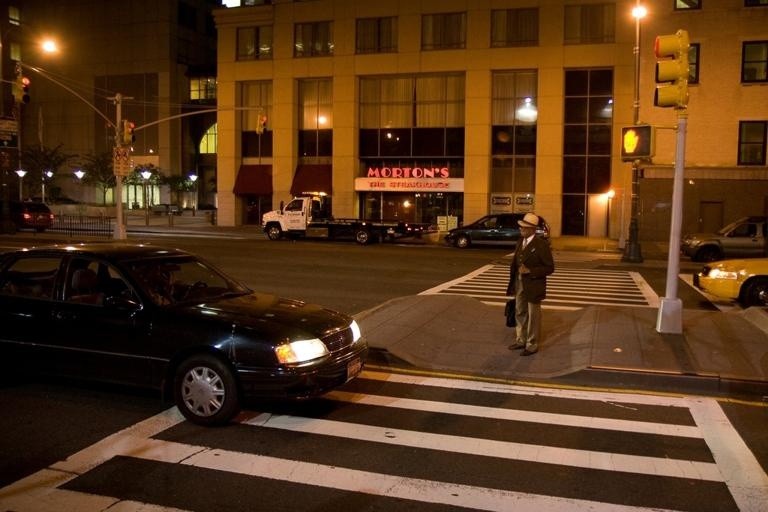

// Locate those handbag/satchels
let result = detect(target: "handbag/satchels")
[504,298,518,327]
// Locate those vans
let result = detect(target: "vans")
[443,212,551,250]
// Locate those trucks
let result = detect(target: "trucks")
[259,193,439,246]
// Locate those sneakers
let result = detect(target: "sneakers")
[509,343,525,350]
[520,349,537,356]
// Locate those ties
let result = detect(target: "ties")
[521,238,528,250]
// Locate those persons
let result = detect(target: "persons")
[504,212,555,356]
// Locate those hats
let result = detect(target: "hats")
[517,212,539,229]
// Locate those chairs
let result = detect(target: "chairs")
[6,268,176,306]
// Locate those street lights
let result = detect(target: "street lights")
[188,174,200,217]
[16,167,87,224]
[140,170,154,227]
[622,2,651,263]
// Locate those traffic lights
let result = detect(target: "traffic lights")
[620,124,656,162]
[15,74,31,105]
[255,113,269,136]
[123,120,136,146]
[654,35,678,106]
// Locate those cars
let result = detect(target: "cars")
[1,240,370,429]
[151,204,183,216]
[680,213,768,307]
[0,201,56,234]
